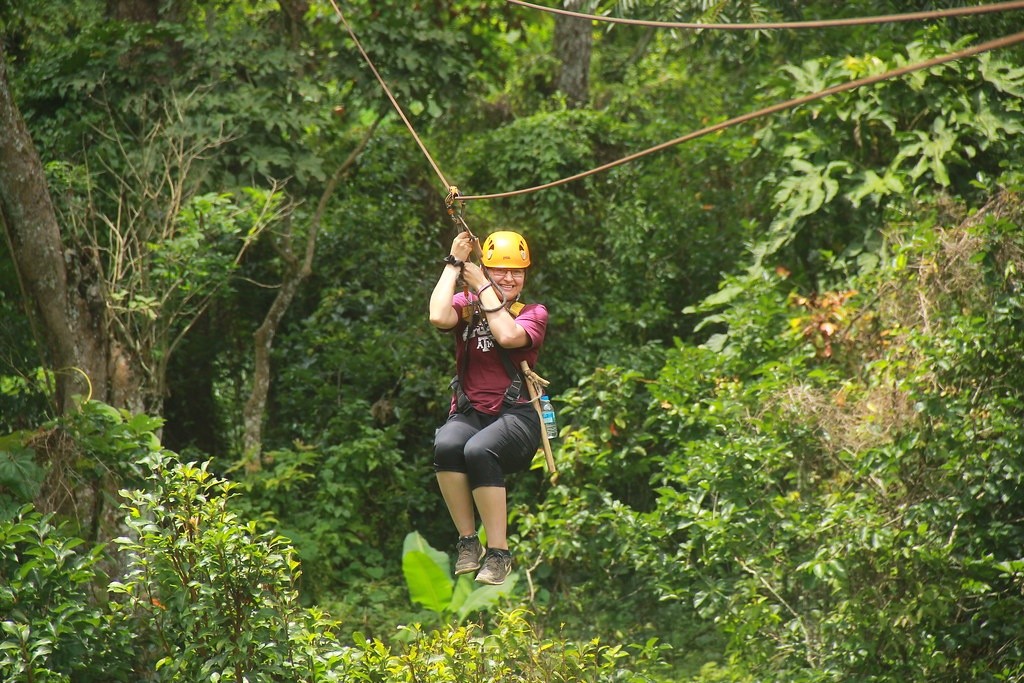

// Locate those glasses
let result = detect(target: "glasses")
[488,267,525,277]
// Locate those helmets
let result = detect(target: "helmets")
[479,230,532,267]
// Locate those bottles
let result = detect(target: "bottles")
[541,395,557,439]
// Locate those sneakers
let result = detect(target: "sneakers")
[474,548,512,585]
[454,533,486,575]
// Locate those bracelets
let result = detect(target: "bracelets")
[444,255,464,270]
[478,282,491,297]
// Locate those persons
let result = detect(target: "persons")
[430,230,548,585]
